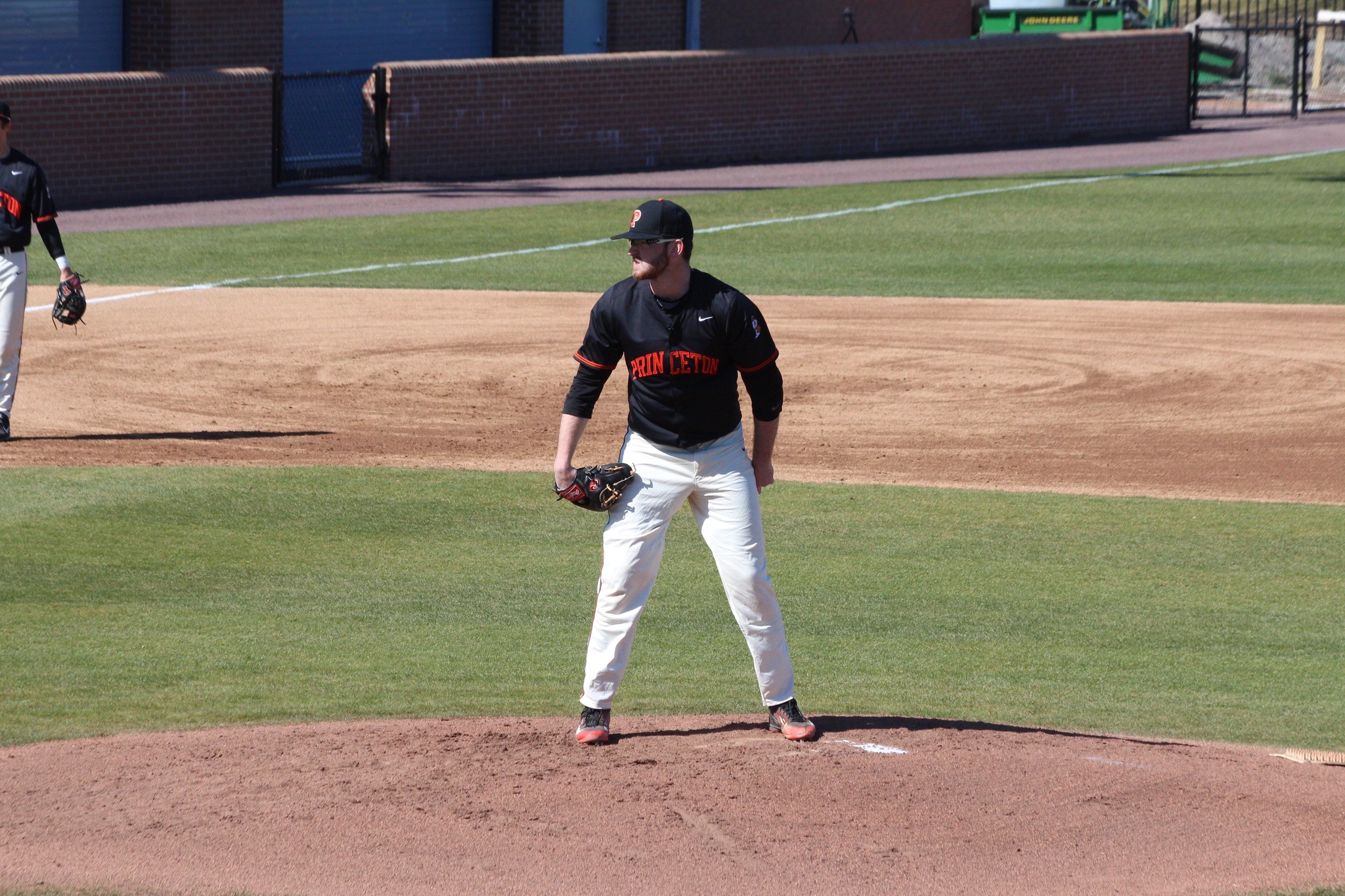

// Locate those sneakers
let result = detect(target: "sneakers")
[768,698,816,740]
[575,706,611,743]
[0,412,11,441]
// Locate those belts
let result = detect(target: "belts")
[0,245,25,255]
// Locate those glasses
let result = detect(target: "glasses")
[628,237,684,246]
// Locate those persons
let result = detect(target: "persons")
[552,198,816,743]
[0,101,90,441]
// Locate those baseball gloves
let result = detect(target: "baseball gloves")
[51,272,87,326]
[553,462,635,513]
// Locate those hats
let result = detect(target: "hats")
[610,198,693,240]
[0,100,11,122]
